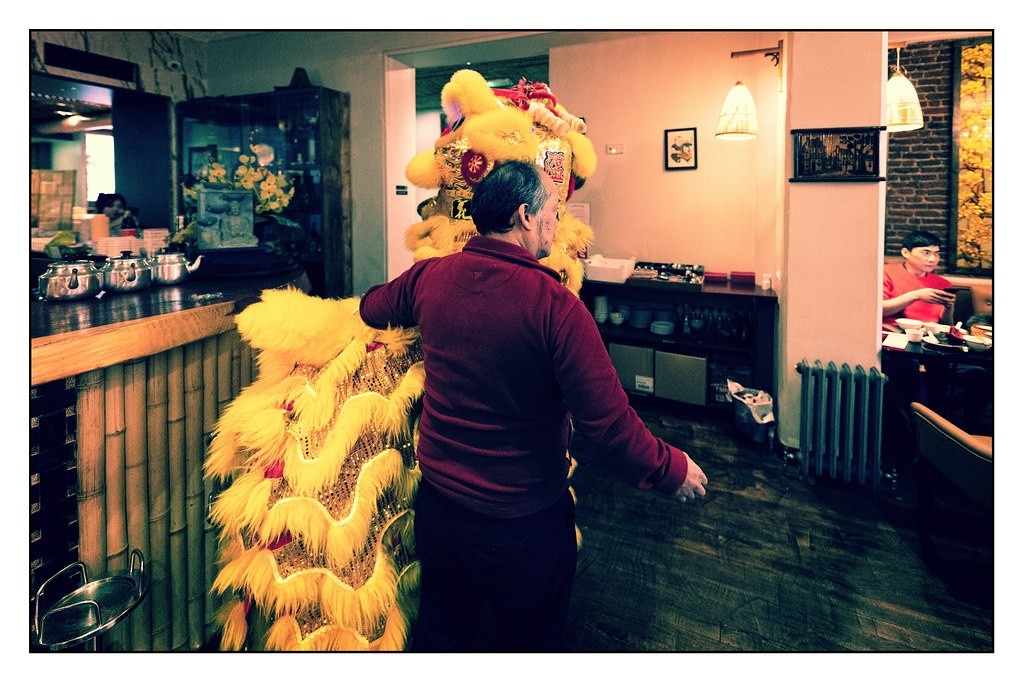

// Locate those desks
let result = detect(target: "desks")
[883,325,993,426]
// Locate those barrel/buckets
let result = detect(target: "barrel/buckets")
[653,304,675,323]
[628,301,656,328]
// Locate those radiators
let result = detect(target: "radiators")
[794,358,887,490]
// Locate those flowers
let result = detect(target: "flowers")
[164,143,303,243]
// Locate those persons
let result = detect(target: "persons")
[359,159,708,652]
[883,230,956,323]
[96,193,143,238]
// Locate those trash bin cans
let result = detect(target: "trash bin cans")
[731,388,772,438]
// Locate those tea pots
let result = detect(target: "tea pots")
[38,254,100,301]
[100,251,148,292]
[148,246,204,283]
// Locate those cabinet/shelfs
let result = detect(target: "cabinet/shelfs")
[175,87,354,301]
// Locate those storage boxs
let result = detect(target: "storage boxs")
[608,342,706,406]
[584,254,705,292]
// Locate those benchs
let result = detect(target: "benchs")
[580,278,778,416]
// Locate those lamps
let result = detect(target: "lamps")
[886,41,924,133]
[714,39,783,140]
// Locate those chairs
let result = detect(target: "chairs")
[909,401,993,531]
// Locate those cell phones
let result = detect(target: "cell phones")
[938,288,959,302]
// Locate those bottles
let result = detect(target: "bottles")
[678,304,750,342]
[72,207,137,242]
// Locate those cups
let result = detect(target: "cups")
[762,280,770,290]
[905,328,924,342]
[763,274,772,290]
[924,323,941,335]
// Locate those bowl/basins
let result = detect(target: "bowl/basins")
[895,318,922,330]
[595,295,629,325]
[962,335,992,350]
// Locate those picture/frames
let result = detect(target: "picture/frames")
[945,37,992,277]
[664,127,698,171]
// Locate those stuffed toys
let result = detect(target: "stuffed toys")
[204,70,596,653]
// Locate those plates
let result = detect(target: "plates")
[939,325,967,334]
[95,228,169,257]
[922,335,970,349]
[650,321,675,335]
